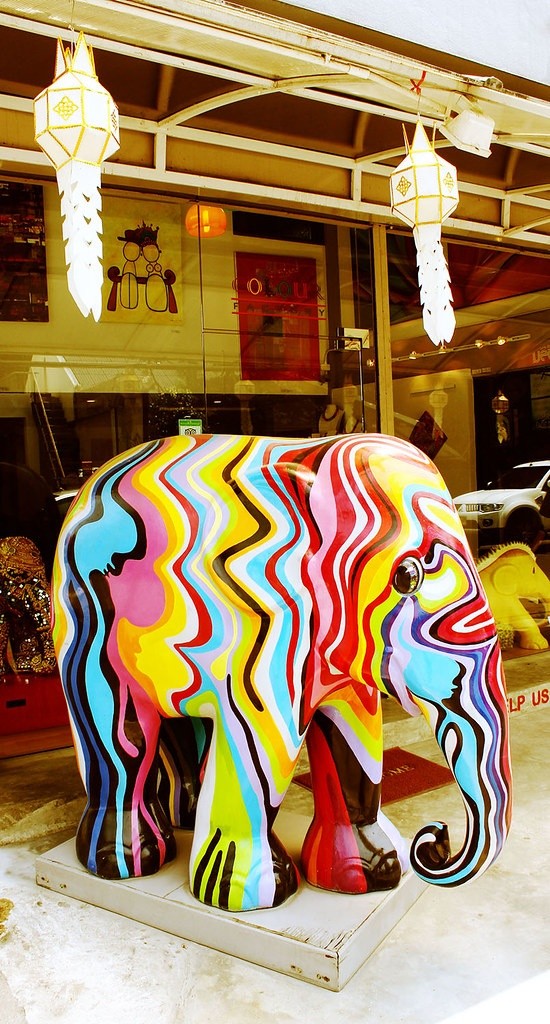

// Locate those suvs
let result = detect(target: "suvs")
[454,458,550,547]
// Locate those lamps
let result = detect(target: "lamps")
[437,110,499,157]
[389,112,461,346]
[32,31,121,326]
[185,202,226,239]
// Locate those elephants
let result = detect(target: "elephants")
[45,431,515,915]
[474,542,550,651]
[0,536,57,678]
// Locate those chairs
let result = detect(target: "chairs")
[1,463,62,584]
